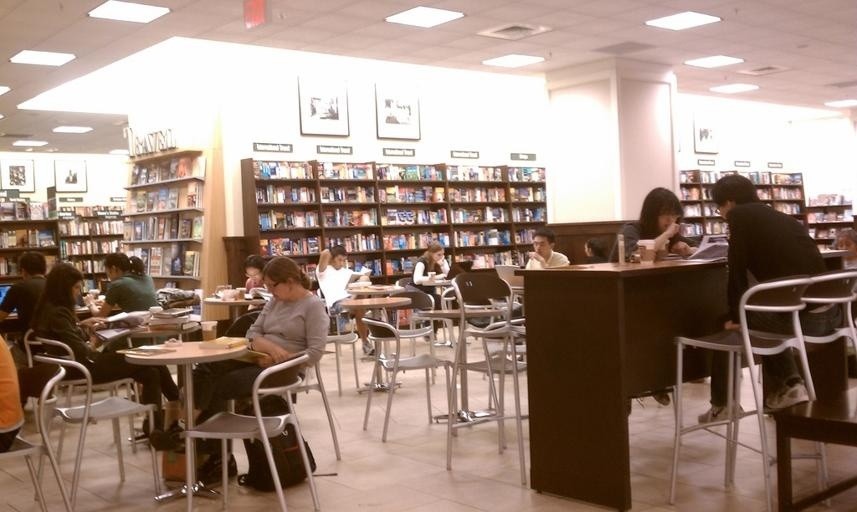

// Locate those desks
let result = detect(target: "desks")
[426,278,471,348]
[128,315,202,339]
[763,388,857,512]
[513,251,850,512]
[203,295,265,320]
[4,300,120,320]
[338,297,412,396]
[123,341,251,504]
[346,285,406,358]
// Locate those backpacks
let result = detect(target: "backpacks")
[236,397,317,494]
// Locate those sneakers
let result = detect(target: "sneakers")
[361,342,375,355]
[196,454,238,483]
[149,428,185,455]
[764,377,810,410]
[697,402,746,425]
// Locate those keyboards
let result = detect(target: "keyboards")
[8,312,17,317]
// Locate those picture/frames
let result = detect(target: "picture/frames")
[692,118,721,155]
[375,83,422,140]
[53,158,88,193]
[297,75,351,137]
[0,158,36,193]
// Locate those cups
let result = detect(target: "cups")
[89,289,99,297]
[428,272,437,282]
[236,288,246,299]
[201,321,218,341]
[637,239,656,264]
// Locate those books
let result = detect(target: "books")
[753,187,771,199]
[60,238,117,258]
[378,183,444,202]
[317,159,373,179]
[259,208,319,230]
[514,206,545,223]
[678,218,729,235]
[199,335,247,351]
[231,351,268,364]
[680,185,715,199]
[516,228,536,244]
[346,269,372,288]
[1,197,52,221]
[376,164,444,180]
[808,195,852,249]
[511,186,545,202]
[679,171,699,182]
[387,253,449,275]
[243,287,268,299]
[344,256,383,277]
[259,233,318,256]
[446,164,502,181]
[700,171,734,184]
[772,173,801,184]
[508,166,544,182]
[1,255,9,275]
[682,204,721,215]
[92,312,149,343]
[518,248,531,267]
[324,207,376,225]
[129,182,201,211]
[775,202,801,215]
[133,156,192,185]
[320,182,373,201]
[126,247,199,276]
[149,308,199,330]
[326,232,379,250]
[115,346,175,356]
[58,220,125,237]
[773,187,803,198]
[454,227,514,245]
[381,229,450,250]
[58,203,123,221]
[451,205,507,222]
[660,232,730,261]
[253,160,315,180]
[255,182,316,202]
[382,207,448,225]
[0,225,58,248]
[455,251,512,266]
[62,259,110,273]
[449,185,505,202]
[44,255,55,276]
[740,171,771,184]
[124,212,203,240]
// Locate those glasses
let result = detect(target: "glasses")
[263,280,285,290]
[715,205,723,217]
[244,270,261,278]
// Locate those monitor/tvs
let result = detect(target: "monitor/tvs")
[0,284,17,312]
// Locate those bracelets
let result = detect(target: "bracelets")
[85,302,95,307]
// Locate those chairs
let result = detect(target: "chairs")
[733,270,857,507]
[444,272,532,487]
[291,361,342,460]
[180,352,321,511]
[360,316,452,444]
[1,362,74,512]
[34,351,162,506]
[440,286,459,350]
[395,277,414,288]
[33,334,139,460]
[23,327,40,369]
[305,331,360,396]
[384,291,436,385]
[668,275,833,512]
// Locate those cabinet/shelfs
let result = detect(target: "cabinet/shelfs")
[240,156,324,290]
[808,199,853,251]
[678,171,704,238]
[446,165,515,271]
[59,220,124,292]
[316,159,385,286]
[124,147,229,324]
[376,163,456,277]
[1,218,59,284]
[509,167,547,267]
[773,173,804,226]
[739,171,773,210]
[701,172,735,235]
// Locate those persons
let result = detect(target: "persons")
[581,239,610,264]
[149,259,330,486]
[409,242,450,344]
[607,187,694,406]
[698,174,841,427]
[525,228,570,270]
[0,251,48,364]
[0,334,25,452]
[244,252,270,294]
[28,263,181,440]
[836,230,857,324]
[80,253,161,320]
[316,245,375,355]
